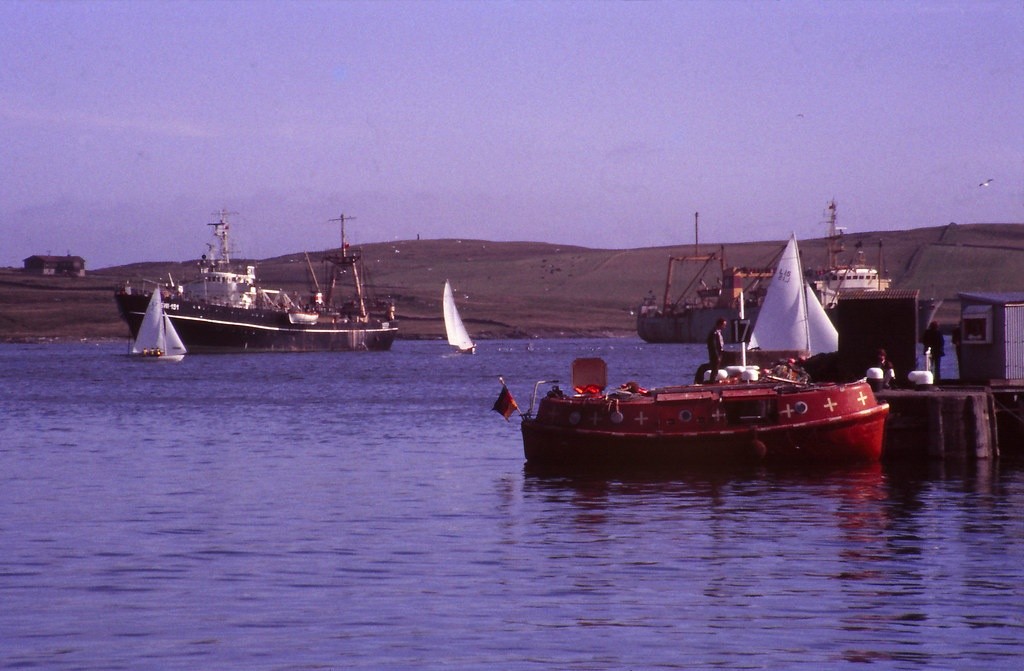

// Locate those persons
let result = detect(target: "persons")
[922,321,945,380]
[716,277,722,287]
[698,279,708,308]
[952,322,961,356]
[648,290,656,305]
[708,318,727,384]
[878,350,895,389]
[142,347,161,356]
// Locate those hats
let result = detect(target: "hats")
[878,349,887,356]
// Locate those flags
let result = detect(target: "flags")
[493,385,518,421]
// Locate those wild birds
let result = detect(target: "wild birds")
[979,178,994,187]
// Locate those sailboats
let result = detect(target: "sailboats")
[127,283,187,363]
[442,279,475,355]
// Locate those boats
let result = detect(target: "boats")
[489,353,888,473]
[637,201,945,344]
[114,210,400,350]
